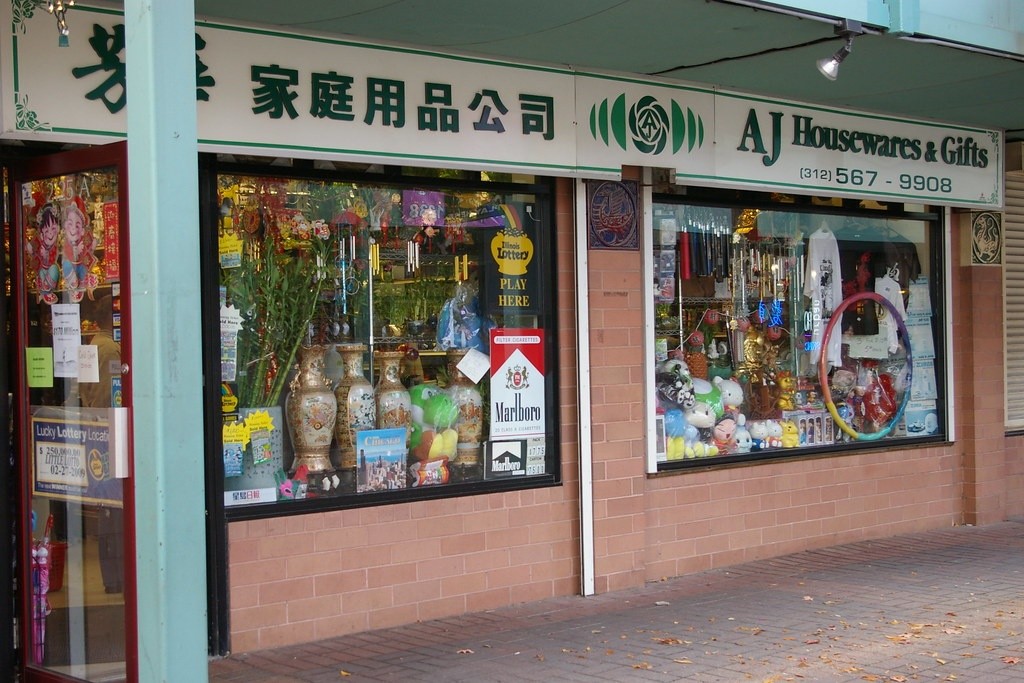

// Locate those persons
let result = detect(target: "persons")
[80,295,123,594]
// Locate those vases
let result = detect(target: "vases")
[441,348,483,469]
[284,341,337,474]
[372,351,411,468]
[333,345,377,470]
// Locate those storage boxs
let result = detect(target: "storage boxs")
[38,539,67,593]
[813,412,824,444]
[806,414,815,445]
[822,412,834,444]
[790,415,808,447]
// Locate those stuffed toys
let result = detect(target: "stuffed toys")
[654,357,799,462]
[406,384,459,461]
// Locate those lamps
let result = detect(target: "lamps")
[815,37,854,81]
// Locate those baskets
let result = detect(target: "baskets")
[685,353,707,379]
[47,541,67,592]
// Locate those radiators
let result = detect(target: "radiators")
[819,215,830,232]
[848,217,866,229]
[878,219,899,235]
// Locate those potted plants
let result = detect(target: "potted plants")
[421,265,449,332]
[218,233,342,490]
[407,276,424,336]
[374,283,405,337]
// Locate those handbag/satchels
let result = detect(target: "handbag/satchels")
[715,274,731,299]
